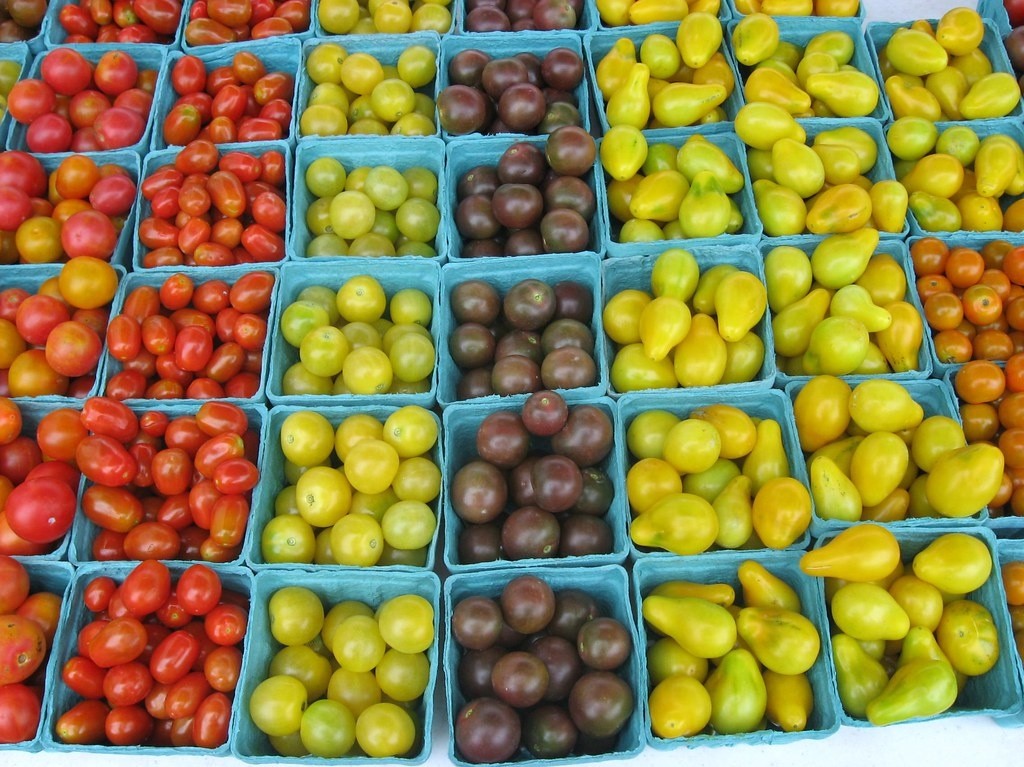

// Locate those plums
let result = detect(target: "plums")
[232,1,640,759]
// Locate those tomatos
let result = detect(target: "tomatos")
[898,230,1024,666]
[0,0,311,759]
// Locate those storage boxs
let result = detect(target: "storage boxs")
[0,0,1022,762]
